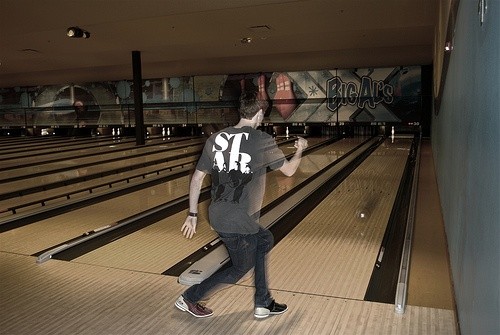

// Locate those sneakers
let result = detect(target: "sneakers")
[174,294,215,318]
[254,300,287,318]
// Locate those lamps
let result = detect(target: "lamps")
[82,31,90,38]
[66,26,83,38]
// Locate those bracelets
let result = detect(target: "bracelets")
[187,210,199,217]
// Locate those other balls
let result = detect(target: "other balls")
[355,207,371,223]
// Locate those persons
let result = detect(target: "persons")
[175,91,308,320]
